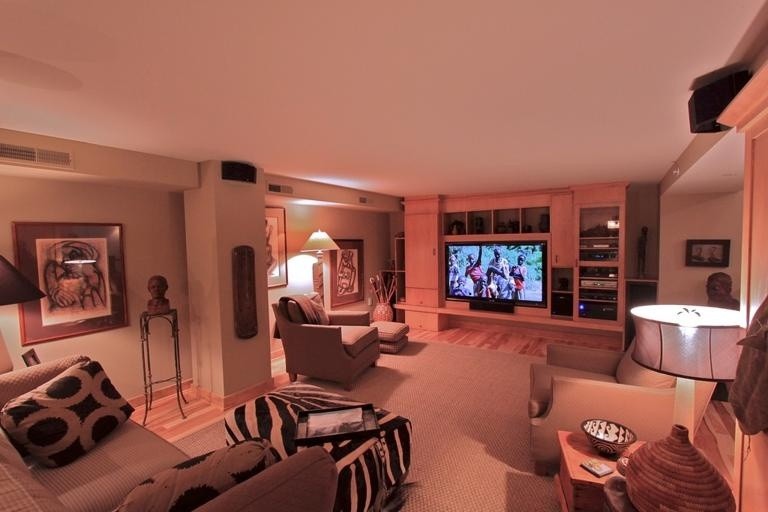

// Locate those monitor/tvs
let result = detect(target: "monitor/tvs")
[445,240,547,308]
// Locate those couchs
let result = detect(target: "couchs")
[0,355,339,512]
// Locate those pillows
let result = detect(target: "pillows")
[110,437,283,512]
[288,293,330,324]
[0,360,135,468]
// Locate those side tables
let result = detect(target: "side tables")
[554,430,647,512]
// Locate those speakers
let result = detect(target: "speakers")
[688,70,751,133]
[551,293,573,316]
[221,161,256,183]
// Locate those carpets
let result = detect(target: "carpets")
[167,339,563,511]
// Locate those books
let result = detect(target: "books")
[293,402,380,445]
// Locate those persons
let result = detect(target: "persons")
[705,272,740,310]
[146,275,171,315]
[449,248,527,300]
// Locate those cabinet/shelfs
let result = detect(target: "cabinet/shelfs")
[393,182,632,351]
[140,309,188,426]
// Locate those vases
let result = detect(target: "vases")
[626,425,736,512]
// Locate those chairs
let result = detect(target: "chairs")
[527,343,718,477]
[272,303,379,391]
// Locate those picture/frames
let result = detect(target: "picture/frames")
[329,239,365,308]
[21,348,41,367]
[264,207,288,288]
[687,240,730,267]
[10,221,130,347]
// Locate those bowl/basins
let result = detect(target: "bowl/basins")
[581,419,637,456]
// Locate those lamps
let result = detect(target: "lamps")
[0,255,46,375]
[629,304,747,443]
[300,228,341,306]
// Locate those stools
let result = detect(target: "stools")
[370,321,409,354]
[222,381,414,512]
